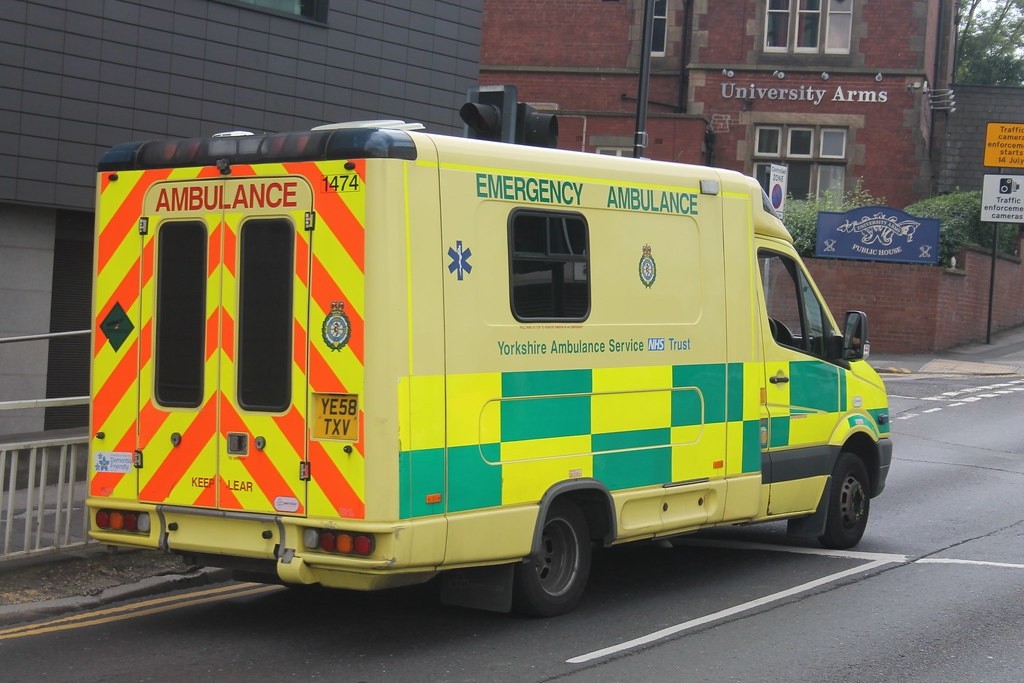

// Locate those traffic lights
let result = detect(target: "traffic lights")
[461,91,505,142]
[516,102,560,149]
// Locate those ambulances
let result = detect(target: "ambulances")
[85,120,893,619]
